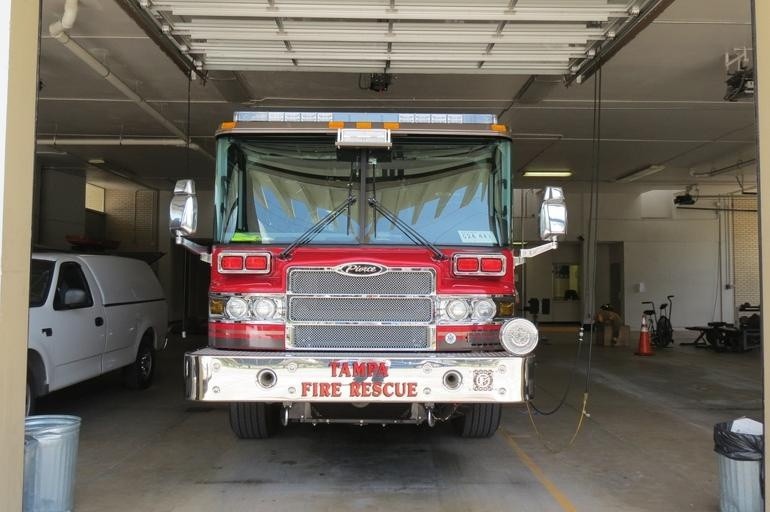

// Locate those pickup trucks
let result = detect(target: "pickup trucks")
[24,248,170,423]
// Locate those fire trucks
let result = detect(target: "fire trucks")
[170,108,569,440]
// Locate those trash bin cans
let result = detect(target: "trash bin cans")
[714,417,765,512]
[23,415,81,512]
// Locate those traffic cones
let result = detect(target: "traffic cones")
[633,314,656,359]
